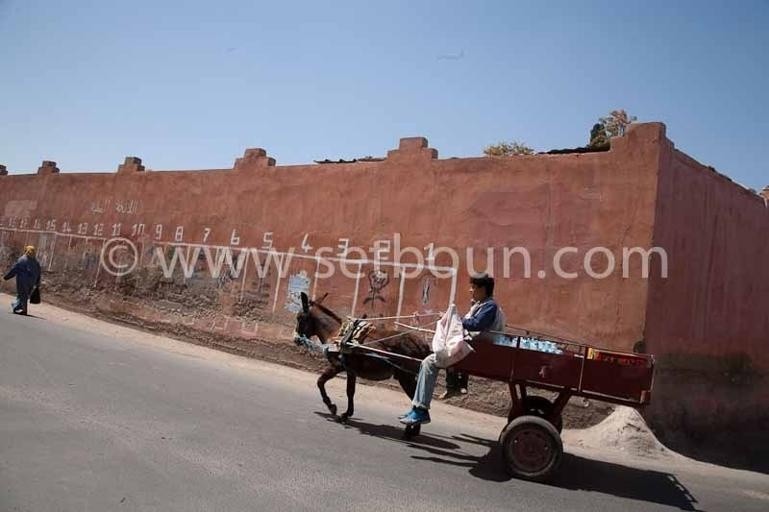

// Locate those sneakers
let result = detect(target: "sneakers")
[439,387,468,399]
[11,304,27,315]
[397,410,430,425]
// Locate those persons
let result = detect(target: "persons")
[397,277,502,425]
[439,273,489,399]
[1,246,41,315]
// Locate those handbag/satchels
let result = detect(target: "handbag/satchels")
[30,287,40,303]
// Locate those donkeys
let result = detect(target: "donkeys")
[293,292,431,441]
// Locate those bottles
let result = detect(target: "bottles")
[512,336,558,353]
